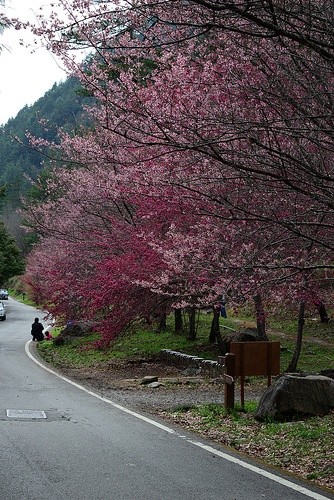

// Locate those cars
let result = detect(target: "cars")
[0,289,8,300]
[0,301,6,321]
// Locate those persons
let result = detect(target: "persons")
[44,331,53,340]
[30,318,45,342]
